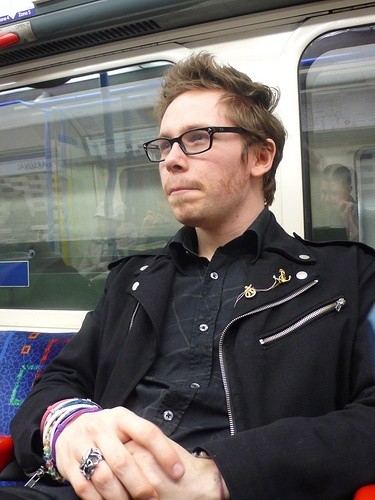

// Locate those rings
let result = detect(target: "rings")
[81,448,102,481]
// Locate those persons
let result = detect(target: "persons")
[318,164,375,249]
[0,184,101,310]
[0,50,375,500]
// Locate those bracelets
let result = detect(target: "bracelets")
[40,397,104,484]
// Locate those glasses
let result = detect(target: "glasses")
[142,125,260,163]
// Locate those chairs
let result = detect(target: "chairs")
[0,331,375,500]
